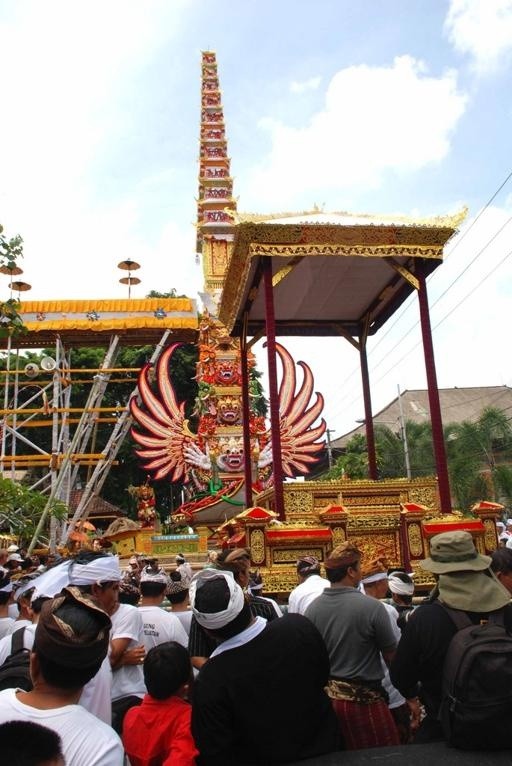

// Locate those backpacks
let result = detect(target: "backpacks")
[434,598,511,752]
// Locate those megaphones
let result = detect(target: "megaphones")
[41,356,56,370]
[24,363,39,378]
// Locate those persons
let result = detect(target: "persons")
[2,519,512,766]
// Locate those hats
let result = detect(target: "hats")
[7,553,26,562]
[419,530,493,575]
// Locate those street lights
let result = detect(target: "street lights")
[356,419,413,480]
